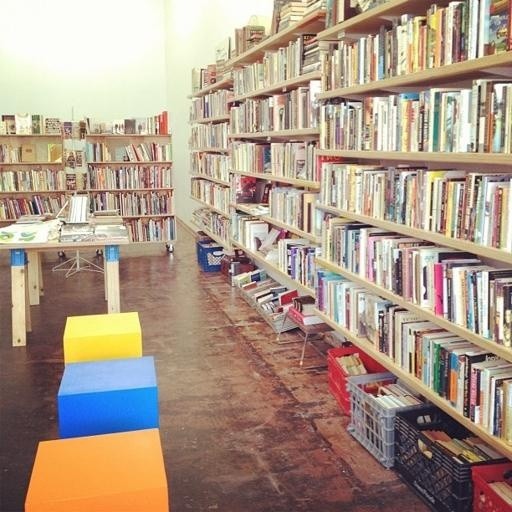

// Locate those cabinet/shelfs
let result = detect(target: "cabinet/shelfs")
[1,113,176,263]
[184,0,512,507]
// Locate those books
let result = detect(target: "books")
[319,330,511,512]
[187,2,328,326]
[316,0,511,443]
[1,111,174,244]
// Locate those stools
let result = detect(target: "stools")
[22,312,170,512]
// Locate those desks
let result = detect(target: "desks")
[1,208,133,351]
